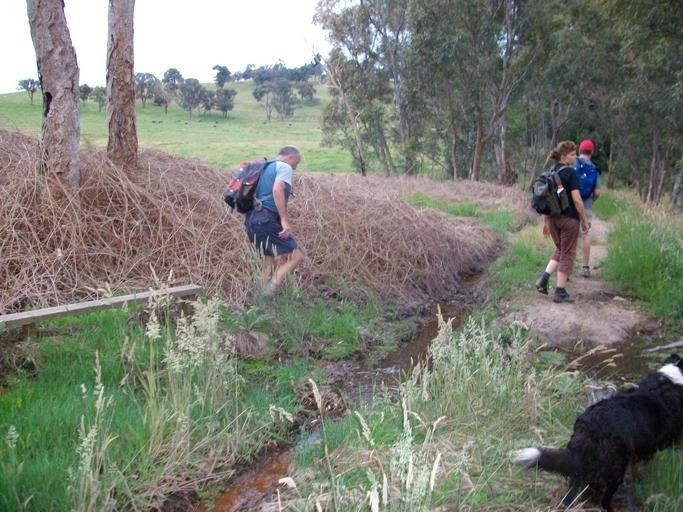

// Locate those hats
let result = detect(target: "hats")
[579,140,594,151]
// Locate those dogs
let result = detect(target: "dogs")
[508,353,683,512]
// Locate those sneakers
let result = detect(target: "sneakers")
[581,268,591,277]
[535,275,548,294]
[553,292,575,303]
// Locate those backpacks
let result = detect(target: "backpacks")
[574,158,601,202]
[531,164,575,218]
[222,157,286,214]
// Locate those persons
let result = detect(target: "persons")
[534,140,589,303]
[243,146,304,297]
[571,139,600,278]
